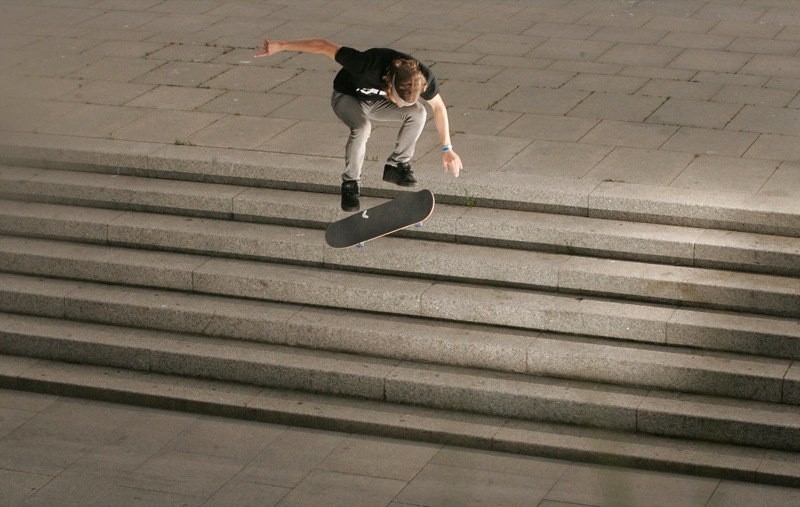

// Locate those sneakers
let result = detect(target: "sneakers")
[382,162,417,187]
[341,180,360,212]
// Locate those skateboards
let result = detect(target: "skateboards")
[324,188,436,250]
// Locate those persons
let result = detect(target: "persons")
[253,34,464,212]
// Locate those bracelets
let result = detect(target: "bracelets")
[441,145,453,152]
